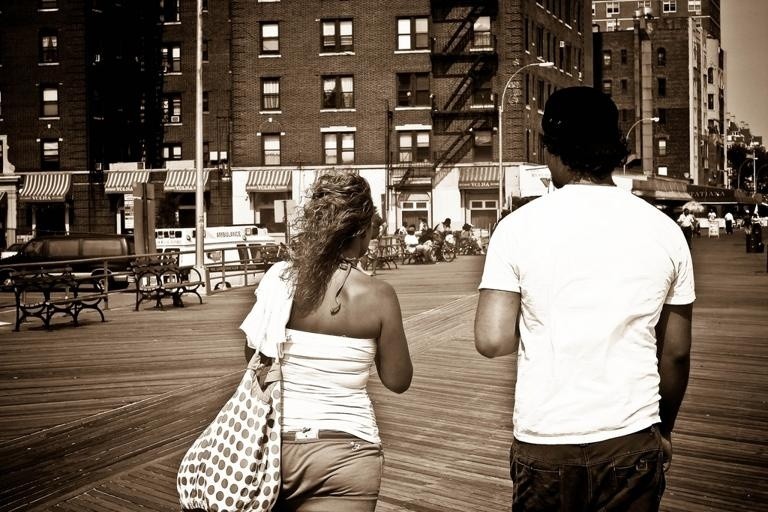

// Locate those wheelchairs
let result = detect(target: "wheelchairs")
[435,230,456,263]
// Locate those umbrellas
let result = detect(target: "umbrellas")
[681,198,704,215]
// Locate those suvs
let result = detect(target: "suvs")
[0,234,128,291]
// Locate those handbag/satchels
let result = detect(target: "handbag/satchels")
[176,312,283,512]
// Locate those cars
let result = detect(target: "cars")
[1,244,25,260]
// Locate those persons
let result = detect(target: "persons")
[237,171,413,512]
[673,207,693,251]
[418,217,428,231]
[459,223,485,255]
[397,220,408,235]
[404,224,434,262]
[473,84,697,512]
[723,209,735,235]
[742,209,751,253]
[707,207,717,223]
[750,210,766,253]
[438,217,455,246]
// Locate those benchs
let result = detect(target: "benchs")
[7,254,208,333]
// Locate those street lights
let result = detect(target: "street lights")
[738,157,758,188]
[500,62,554,221]
[623,117,660,191]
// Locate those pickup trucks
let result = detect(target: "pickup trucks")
[187,241,281,294]
